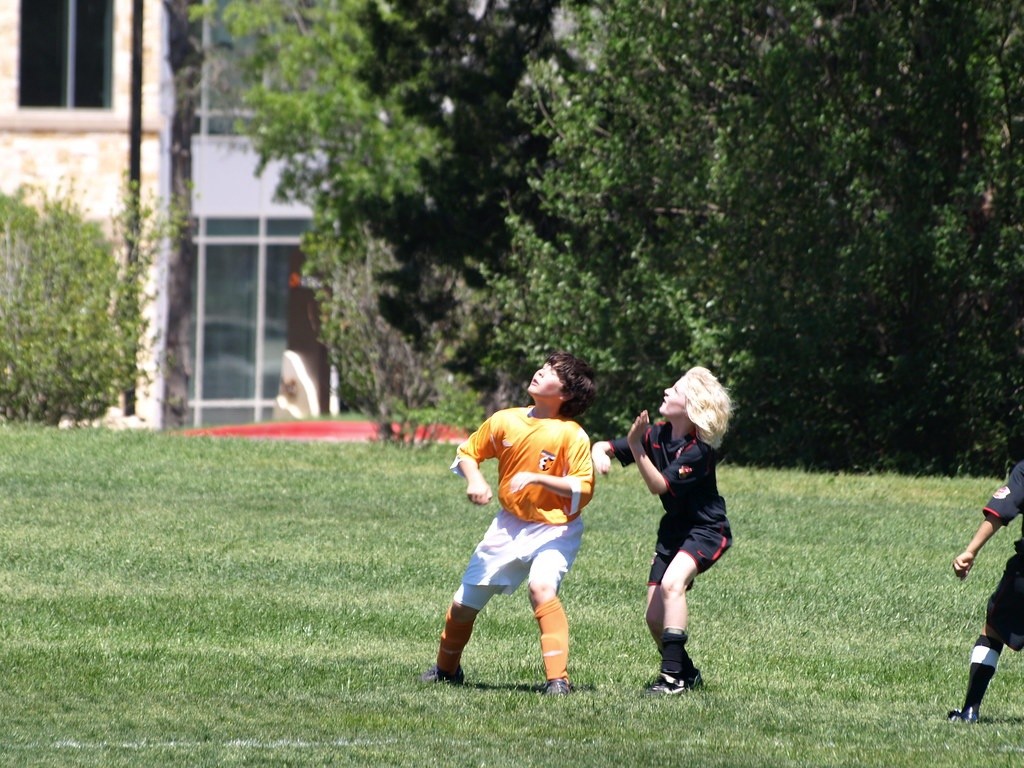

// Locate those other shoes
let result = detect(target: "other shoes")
[420,664,464,685]
[544,678,570,696]
[949,710,979,725]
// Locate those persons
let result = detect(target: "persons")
[424,353,596,696]
[947,460,1024,724]
[592,365,733,696]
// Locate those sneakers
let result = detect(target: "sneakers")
[682,669,702,690]
[647,668,685,698]
